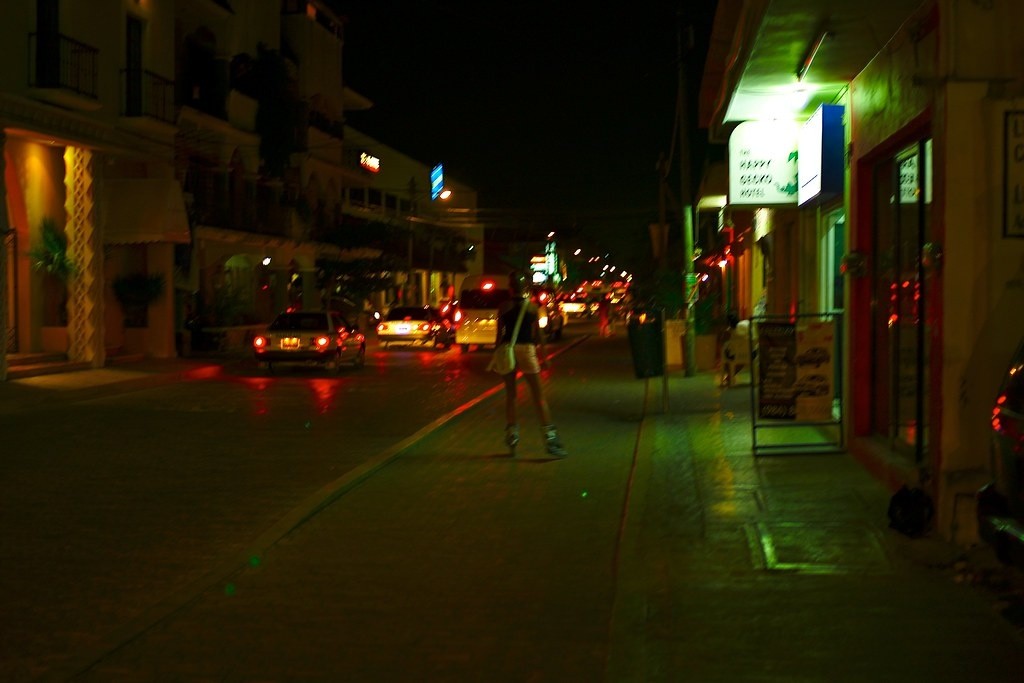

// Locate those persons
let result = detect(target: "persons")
[485,271,570,458]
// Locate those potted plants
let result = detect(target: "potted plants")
[625,259,726,371]
[22,216,114,326]
[110,270,166,327]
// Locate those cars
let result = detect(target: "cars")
[972,357,1024,570]
[377,306,440,350]
[796,345,830,369]
[254,310,367,378]
[791,373,830,398]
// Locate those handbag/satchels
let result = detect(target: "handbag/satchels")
[487,346,516,375]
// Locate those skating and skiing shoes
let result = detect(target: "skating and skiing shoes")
[541,427,566,461]
[506,427,519,459]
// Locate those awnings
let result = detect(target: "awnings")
[102,178,191,246]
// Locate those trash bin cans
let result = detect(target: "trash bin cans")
[628,318,667,380]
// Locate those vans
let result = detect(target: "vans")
[455,273,564,353]
